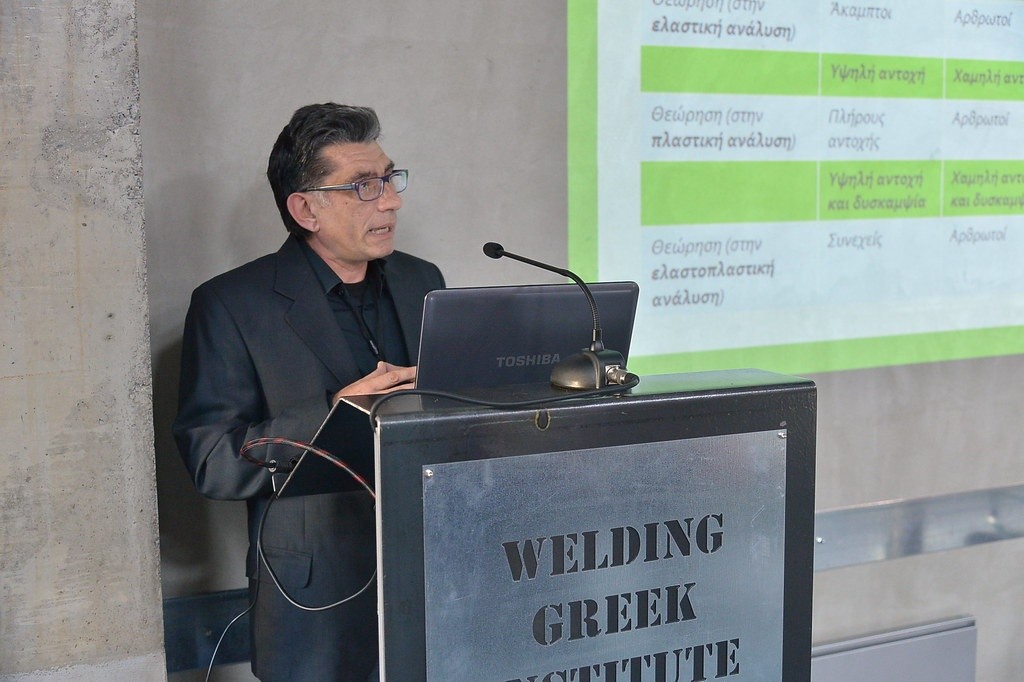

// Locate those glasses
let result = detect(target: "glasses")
[299,170,408,201]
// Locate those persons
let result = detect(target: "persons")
[173,103,446,682]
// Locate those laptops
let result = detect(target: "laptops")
[413,282,640,392]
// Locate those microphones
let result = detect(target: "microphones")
[484,242,628,389]
